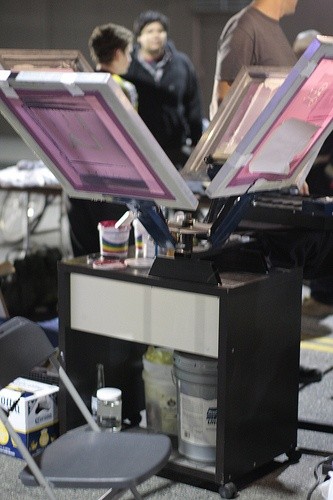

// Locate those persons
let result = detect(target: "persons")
[88,23,140,112]
[292,30,333,340]
[208,0,297,122]
[124,11,203,164]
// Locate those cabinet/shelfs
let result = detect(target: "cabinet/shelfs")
[60,258,306,500]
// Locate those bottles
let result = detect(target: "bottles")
[96,388,123,433]
[141,346,180,438]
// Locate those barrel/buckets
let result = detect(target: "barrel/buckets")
[175,348,216,462]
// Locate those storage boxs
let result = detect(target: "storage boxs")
[0,377,60,459]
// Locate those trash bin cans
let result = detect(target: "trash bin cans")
[143,354,180,442]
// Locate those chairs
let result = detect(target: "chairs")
[0,318,171,500]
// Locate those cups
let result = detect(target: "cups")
[171,228,194,254]
[97,223,131,265]
[132,218,155,261]
[158,226,179,260]
